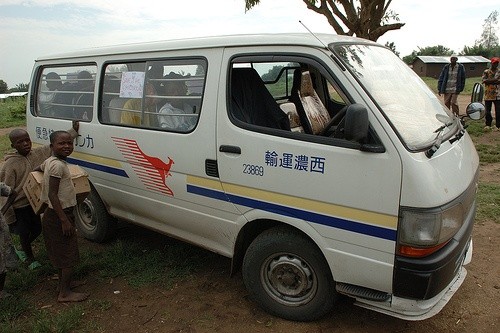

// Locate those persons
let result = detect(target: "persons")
[39,68,201,134]
[32,130,90,303]
[0,119,82,273]
[481,56,500,131]
[436,53,466,119]
[0,181,17,301]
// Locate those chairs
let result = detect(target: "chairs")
[55,83,202,125]
[229,69,332,133]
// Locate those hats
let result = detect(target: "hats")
[491,56,500,63]
[451,53,457,57]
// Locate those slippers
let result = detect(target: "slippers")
[28,260,42,272]
[14,245,28,263]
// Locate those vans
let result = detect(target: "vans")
[25,30,488,323]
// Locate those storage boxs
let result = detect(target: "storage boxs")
[22,165,90,215]
